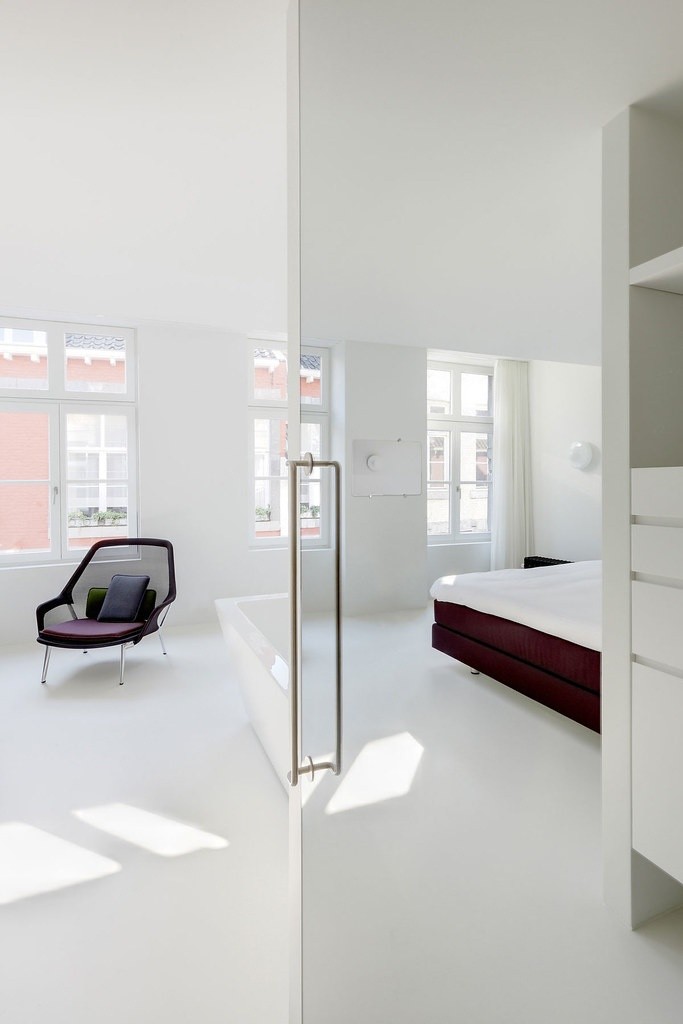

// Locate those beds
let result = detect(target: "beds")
[430,560,602,739]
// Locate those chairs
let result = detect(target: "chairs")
[35,538,177,685]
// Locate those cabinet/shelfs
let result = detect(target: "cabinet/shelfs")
[602,81,683,937]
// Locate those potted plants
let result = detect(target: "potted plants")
[256,506,272,531]
[67,510,127,528]
[301,505,321,529]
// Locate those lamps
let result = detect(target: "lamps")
[565,441,593,468]
